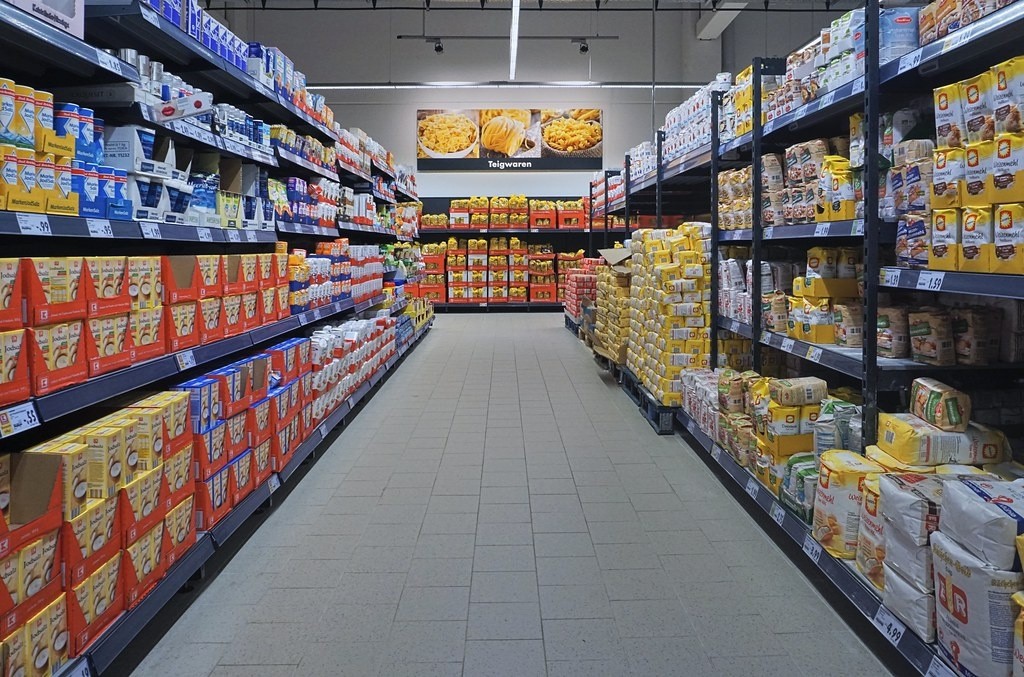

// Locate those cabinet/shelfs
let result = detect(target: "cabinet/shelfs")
[675,56,756,458]
[369,157,397,385]
[711,0,1023,572]
[0,0,218,677]
[49,0,282,548]
[394,184,436,359]
[418,227,636,312]
[335,158,381,425]
[272,92,355,485]
[588,89,717,257]
[802,0,1023,677]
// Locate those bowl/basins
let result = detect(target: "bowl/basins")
[417,113,478,159]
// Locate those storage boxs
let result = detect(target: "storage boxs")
[420,82,712,303]
[718,0,1023,493]
[0,0,436,677]
[561,222,713,408]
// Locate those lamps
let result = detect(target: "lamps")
[434,41,443,54]
[579,43,587,53]
